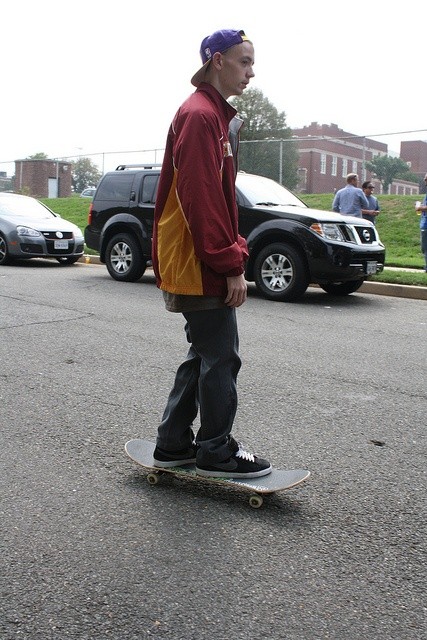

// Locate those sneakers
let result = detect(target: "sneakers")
[195,448,272,478]
[152,441,199,468]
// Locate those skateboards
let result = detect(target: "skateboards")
[124,438,311,509]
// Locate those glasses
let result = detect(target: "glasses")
[365,187,375,188]
[424,178,427,181]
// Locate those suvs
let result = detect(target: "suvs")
[84,163,385,300]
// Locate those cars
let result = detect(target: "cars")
[0,193,84,264]
[80,188,96,197]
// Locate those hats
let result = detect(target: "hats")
[191,30,252,88]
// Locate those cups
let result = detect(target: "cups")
[416,202,422,215]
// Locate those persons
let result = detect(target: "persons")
[331,172,370,219]
[415,173,427,272]
[361,180,380,224]
[147,27,273,479]
